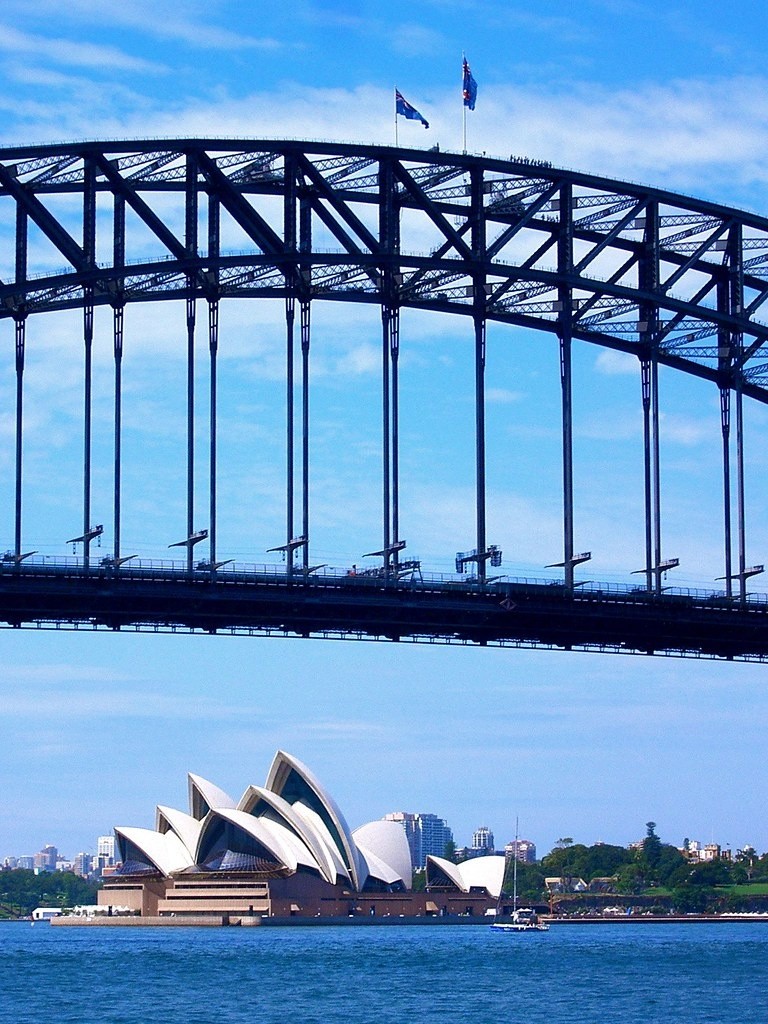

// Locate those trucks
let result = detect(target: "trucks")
[485,908,501,916]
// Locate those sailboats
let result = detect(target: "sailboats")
[489,814,552,933]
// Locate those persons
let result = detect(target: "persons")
[510,154,552,168]
[541,214,558,223]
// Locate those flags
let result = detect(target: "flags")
[462,58,477,111]
[396,89,429,129]
